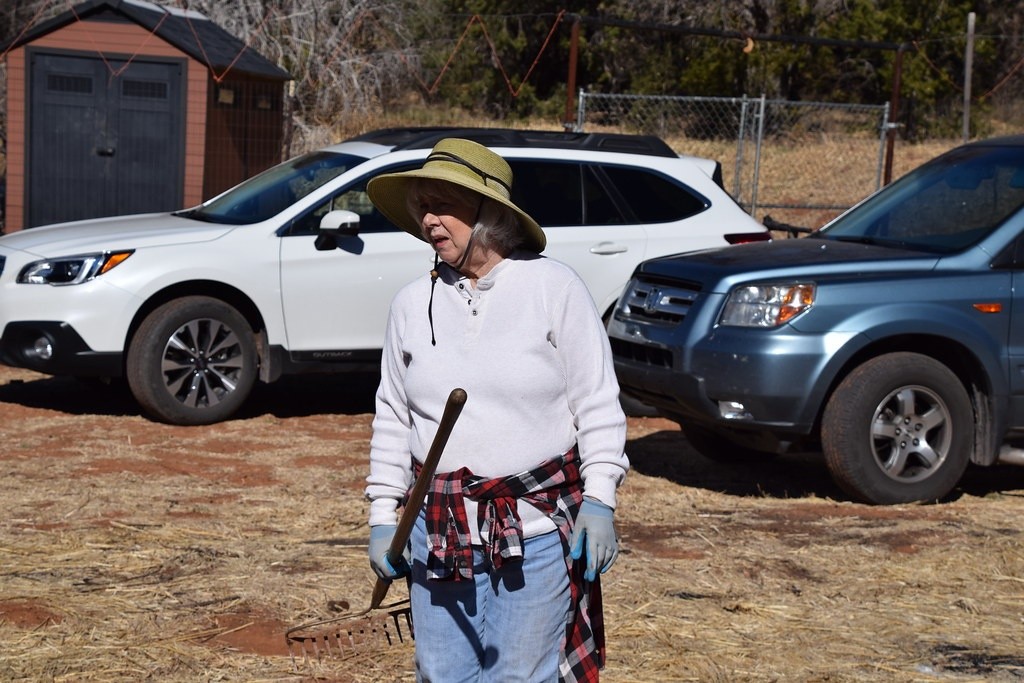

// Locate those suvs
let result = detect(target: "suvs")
[605,130,1023,506]
[0,127,774,426]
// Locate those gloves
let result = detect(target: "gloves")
[570,496,618,582]
[369,526,411,579]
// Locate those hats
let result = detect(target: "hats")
[366,138,547,255]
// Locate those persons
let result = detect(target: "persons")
[362,135,629,683]
